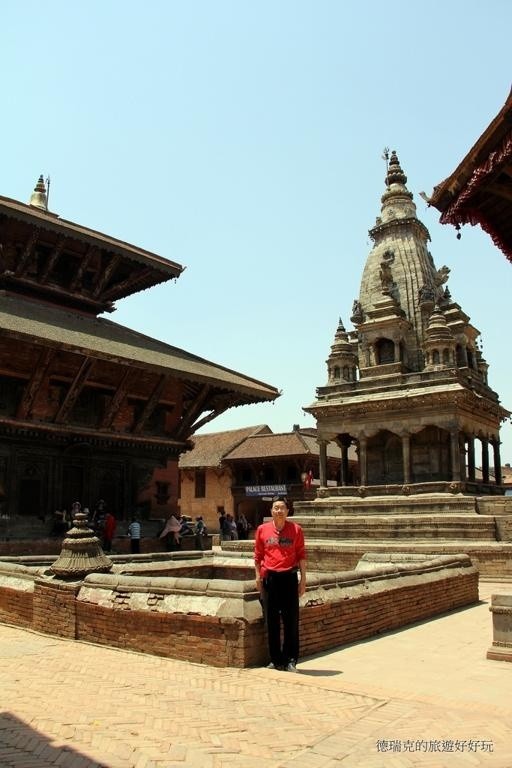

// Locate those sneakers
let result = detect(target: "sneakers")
[266,661,282,671]
[284,658,302,673]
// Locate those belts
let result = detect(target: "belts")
[268,566,298,576]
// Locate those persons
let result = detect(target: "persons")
[51,497,119,552]
[219,509,251,542]
[158,512,208,551]
[128,517,141,554]
[254,496,307,674]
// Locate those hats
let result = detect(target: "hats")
[72,501,82,510]
[97,499,107,507]
